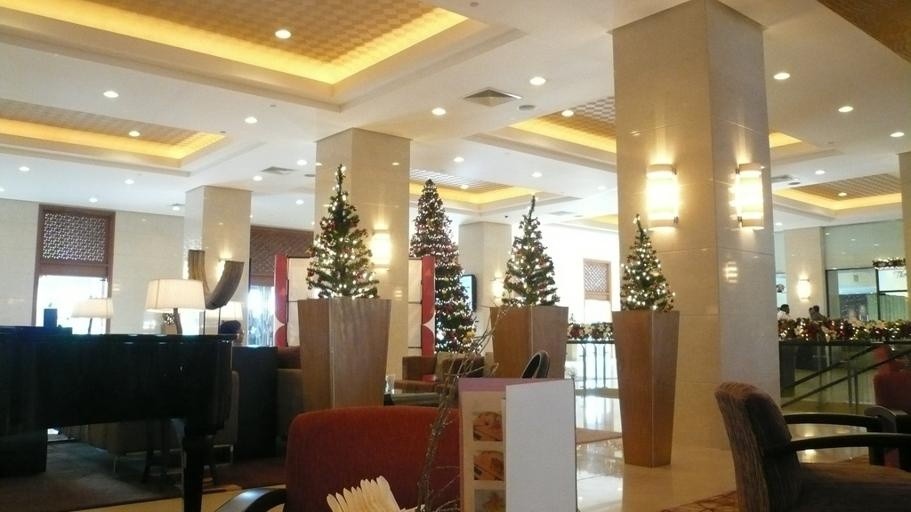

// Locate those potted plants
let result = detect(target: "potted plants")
[296,163,391,411]
[610,213,681,469]
[489,192,569,376]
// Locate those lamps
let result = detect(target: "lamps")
[645,163,678,229]
[73,297,112,335]
[735,161,767,229]
[144,278,206,334]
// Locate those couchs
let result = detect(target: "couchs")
[393,350,485,398]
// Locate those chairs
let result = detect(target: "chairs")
[713,369,911,510]
[214,406,461,511]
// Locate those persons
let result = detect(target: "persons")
[776,304,795,320]
[809,305,826,321]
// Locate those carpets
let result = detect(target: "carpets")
[1,440,285,511]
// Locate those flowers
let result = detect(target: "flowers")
[778,315,910,344]
[568,321,614,341]
